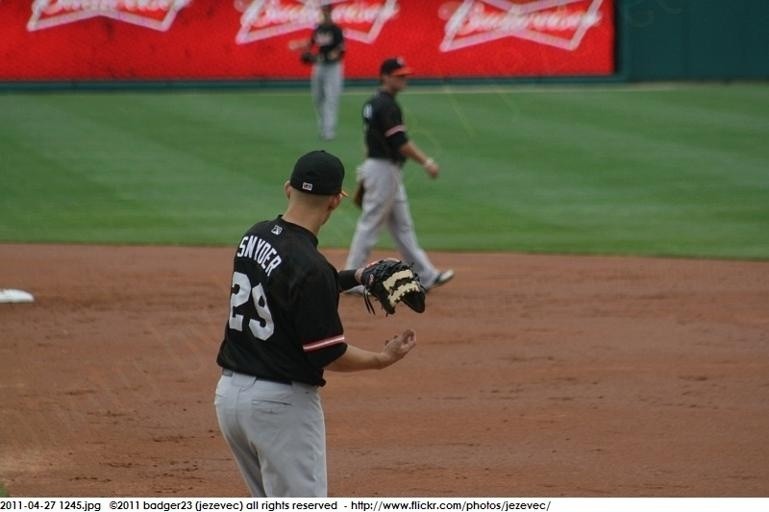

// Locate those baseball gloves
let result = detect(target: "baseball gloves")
[362,257,426,316]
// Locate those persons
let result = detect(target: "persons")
[343,55,456,296]
[300,1,345,140]
[216,149,416,497]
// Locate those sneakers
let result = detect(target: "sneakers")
[424,269,455,293]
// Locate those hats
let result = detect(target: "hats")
[291,150,349,198]
[381,56,414,76]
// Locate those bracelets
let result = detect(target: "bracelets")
[423,156,434,169]
